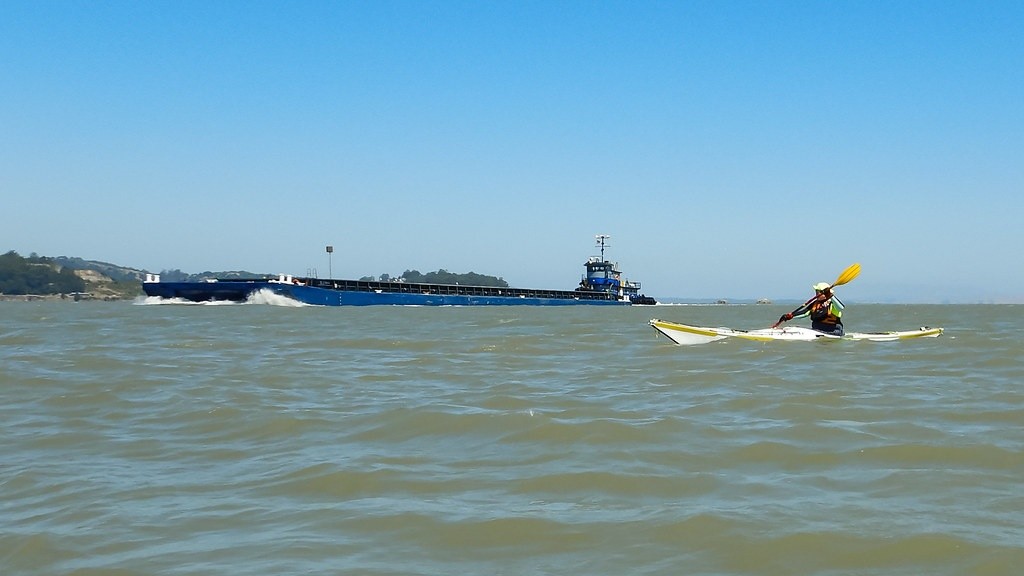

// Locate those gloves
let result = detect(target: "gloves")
[786,313,794,319]
[823,288,831,297]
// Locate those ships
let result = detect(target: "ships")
[141,232,659,309]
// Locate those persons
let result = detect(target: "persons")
[787,282,846,336]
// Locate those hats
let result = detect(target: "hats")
[812,282,833,293]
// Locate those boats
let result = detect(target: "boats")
[650,316,946,346]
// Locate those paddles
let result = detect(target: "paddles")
[772,262,861,329]
